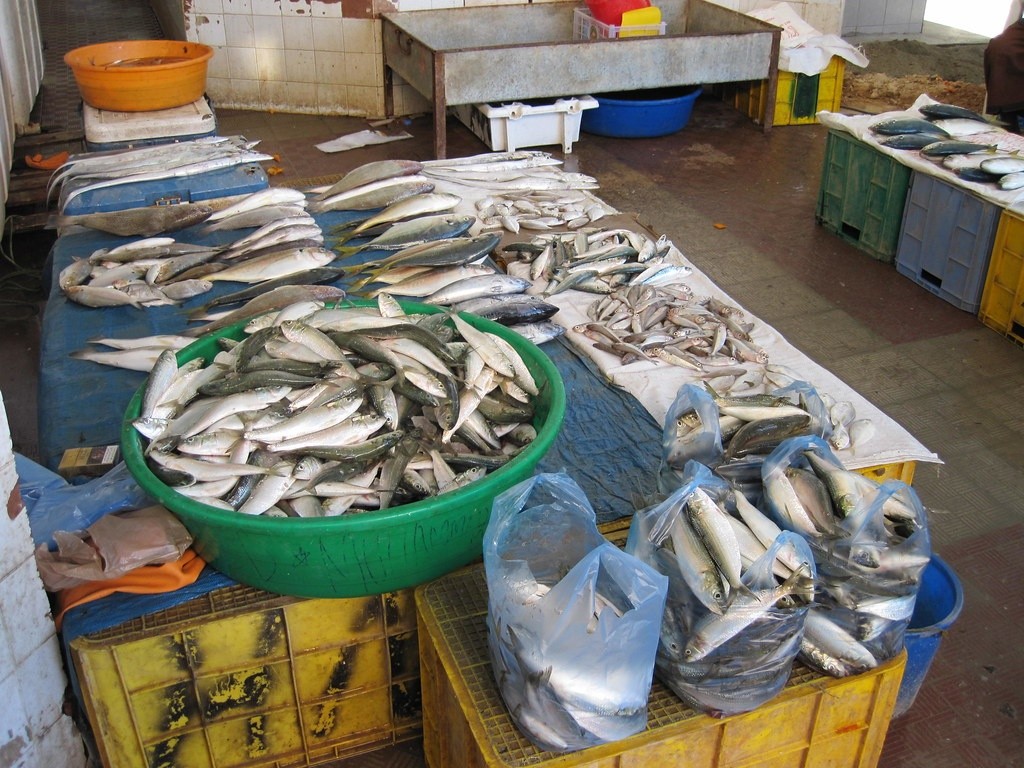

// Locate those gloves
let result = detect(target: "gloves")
[25,151,69,170]
[55,548,205,633]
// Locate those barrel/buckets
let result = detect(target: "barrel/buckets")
[891,550,964,719]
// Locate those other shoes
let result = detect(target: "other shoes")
[1008,110,1024,135]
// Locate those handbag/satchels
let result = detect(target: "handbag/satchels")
[755,435,931,678]
[483,468,669,752]
[655,381,835,508]
[625,459,817,715]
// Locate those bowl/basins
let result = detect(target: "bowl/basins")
[63,40,214,112]
[128,287,566,597]
[575,84,706,139]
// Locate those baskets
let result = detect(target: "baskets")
[572,8,666,38]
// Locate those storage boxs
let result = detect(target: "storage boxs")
[68,462,916,767]
[450,93,599,153]
[720,54,846,127]
[81,96,217,153]
[58,134,271,216]
[815,127,1024,347]
[572,9,668,41]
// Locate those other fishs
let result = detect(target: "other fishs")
[56,102,1024,751]
